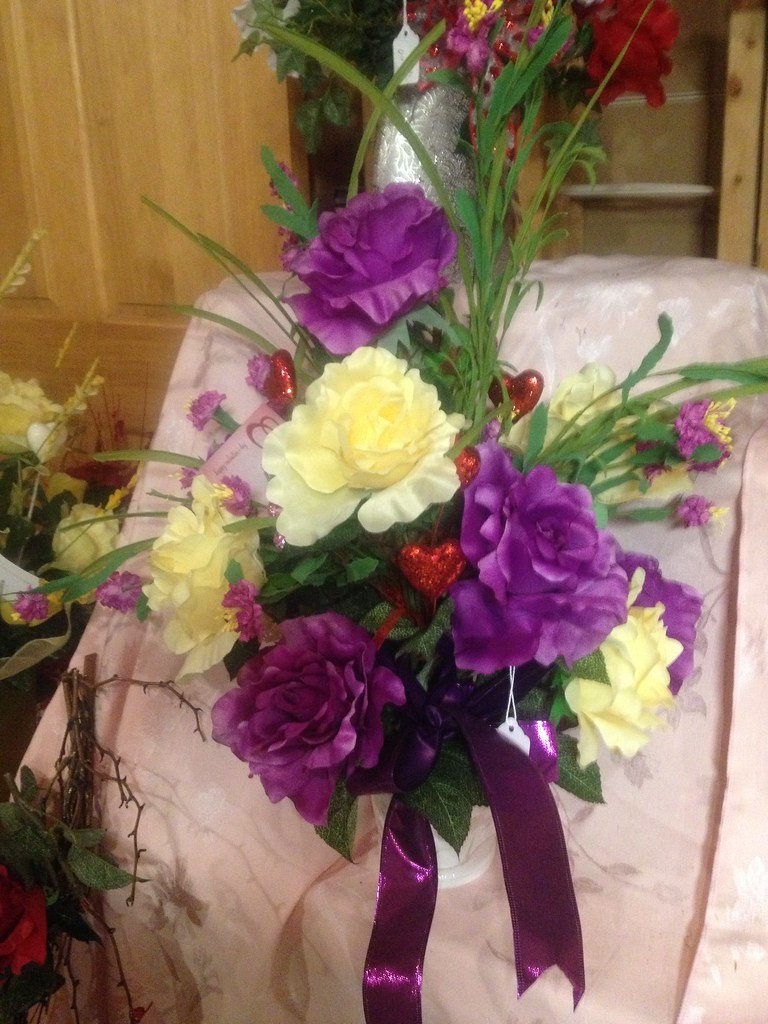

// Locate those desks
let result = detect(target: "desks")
[7,250,767,1024]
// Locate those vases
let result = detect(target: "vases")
[351,55,467,216]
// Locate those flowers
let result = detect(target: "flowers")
[3,226,147,684]
[81,9,767,858]
[236,1,692,167]
